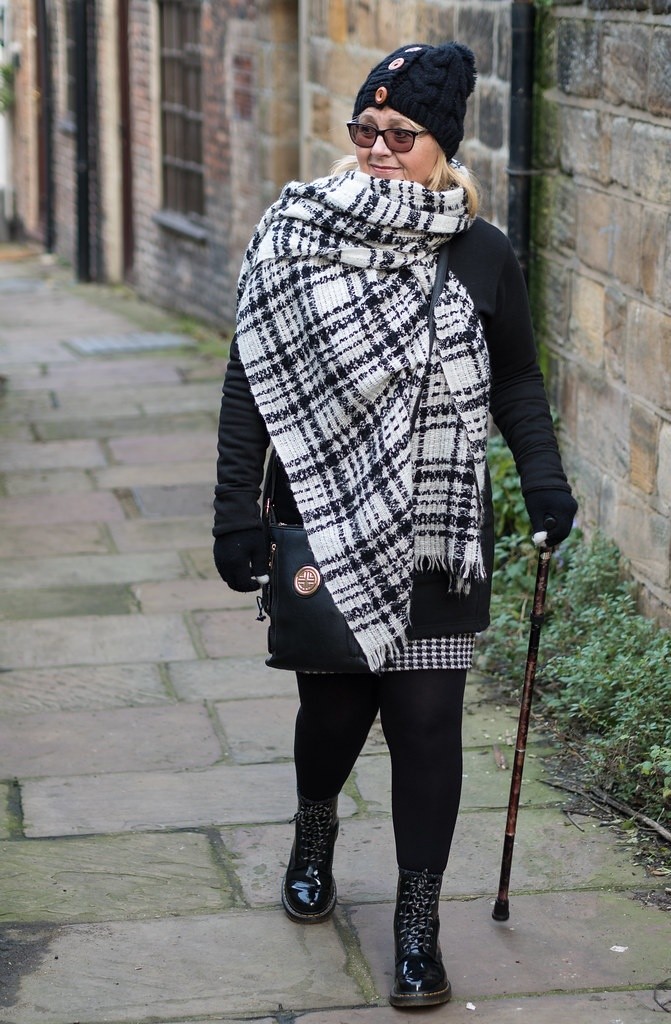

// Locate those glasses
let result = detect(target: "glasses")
[347,117,428,152]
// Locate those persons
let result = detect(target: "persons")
[211,37,577,1009]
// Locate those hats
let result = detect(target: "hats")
[353,40,475,162]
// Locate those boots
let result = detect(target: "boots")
[392,867,452,1008]
[283,796,345,922]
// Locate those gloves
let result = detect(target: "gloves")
[524,489,579,548]
[212,525,267,593]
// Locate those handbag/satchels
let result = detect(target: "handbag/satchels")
[267,520,377,672]
